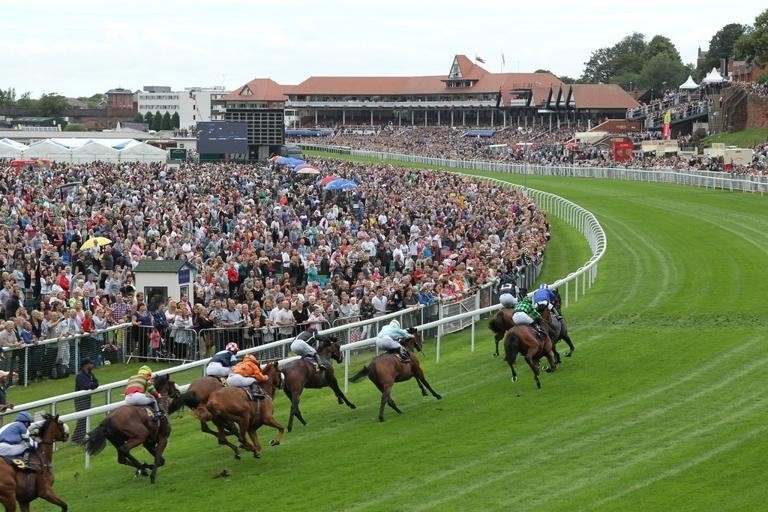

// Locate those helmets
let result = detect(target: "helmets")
[391,320,400,327]
[226,342,240,352]
[308,327,317,334]
[540,283,548,290]
[523,296,532,303]
[16,412,34,422]
[138,366,152,376]
[243,354,256,362]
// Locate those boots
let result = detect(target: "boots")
[531,321,547,338]
[315,353,326,369]
[551,306,563,320]
[251,383,265,398]
[153,401,165,417]
[18,450,32,472]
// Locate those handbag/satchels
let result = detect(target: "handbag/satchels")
[52,364,69,378]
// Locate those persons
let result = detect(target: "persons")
[281,68,767,196]
[0,154,551,361]
[69,358,100,445]
[0,370,14,412]
[125,366,163,420]
[0,411,38,471]
[205,340,238,377]
[226,355,268,400]
[289,325,326,370]
[534,282,554,310]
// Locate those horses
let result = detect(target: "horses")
[500,300,559,393]
[346,326,443,424]
[167,374,240,438]
[190,359,285,462]
[486,286,529,358]
[279,336,357,433]
[0,412,69,512]
[78,371,182,485]
[545,286,575,366]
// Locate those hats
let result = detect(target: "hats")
[81,356,95,365]
[0,370,10,377]
